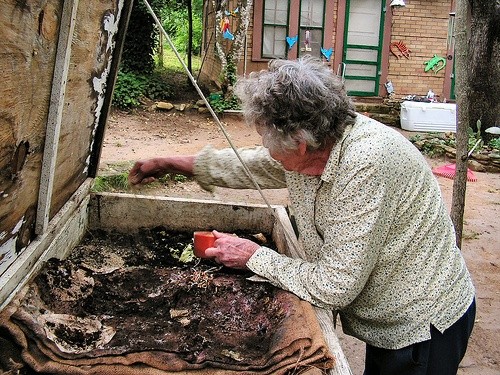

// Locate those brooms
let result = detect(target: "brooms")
[432,137,482,183]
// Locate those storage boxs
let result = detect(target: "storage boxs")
[400,101,456,133]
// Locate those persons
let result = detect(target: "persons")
[127,55,476,375]
